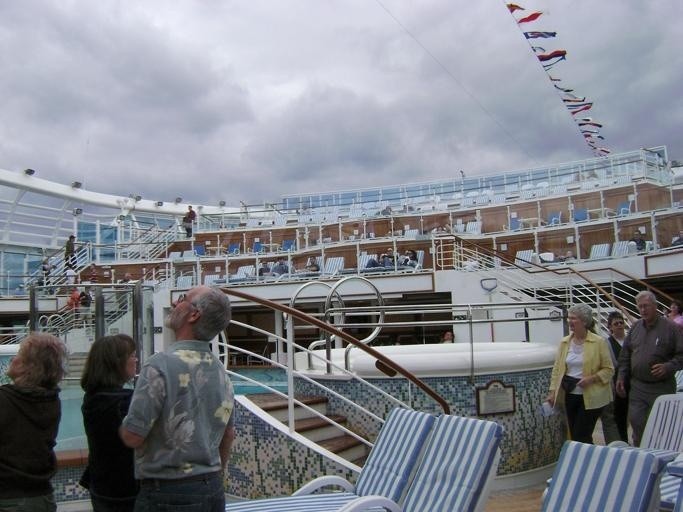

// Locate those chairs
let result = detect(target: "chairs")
[277,251,425,280]
[247,200,389,228]
[462,234,680,269]
[506,202,632,230]
[191,239,296,257]
[412,193,547,211]
[535,440,658,511]
[537,392,682,504]
[161,250,293,291]
[218,404,437,512]
[318,221,482,241]
[339,412,506,512]
[657,454,683,512]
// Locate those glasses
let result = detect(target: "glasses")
[177,293,196,308]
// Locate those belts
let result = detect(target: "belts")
[138,470,225,488]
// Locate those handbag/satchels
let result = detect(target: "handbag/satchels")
[561,374,581,391]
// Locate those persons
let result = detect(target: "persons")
[0,333,70,512]
[670,232,683,249]
[361,221,452,270]
[369,330,456,345]
[254,254,321,278]
[118,284,235,511]
[78,333,139,512]
[627,230,646,251]
[546,291,683,445]
[181,205,197,238]
[37,234,131,338]
